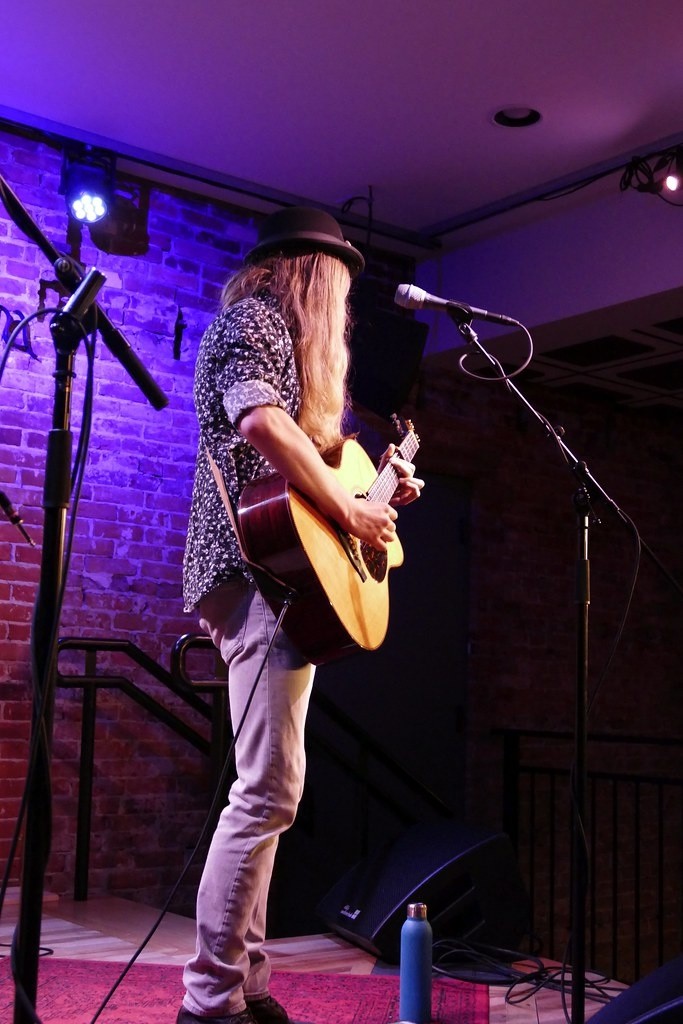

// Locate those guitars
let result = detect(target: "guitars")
[237,413,422,665]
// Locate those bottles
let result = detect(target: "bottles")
[399,903,434,1024]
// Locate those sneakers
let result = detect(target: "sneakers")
[176,1005,258,1024]
[246,995,290,1024]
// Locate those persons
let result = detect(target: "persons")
[173,204,426,1024]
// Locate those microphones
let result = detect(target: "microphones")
[394,284,520,328]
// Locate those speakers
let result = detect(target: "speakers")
[586,956,683,1024]
[319,813,535,960]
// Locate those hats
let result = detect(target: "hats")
[241,205,366,281]
